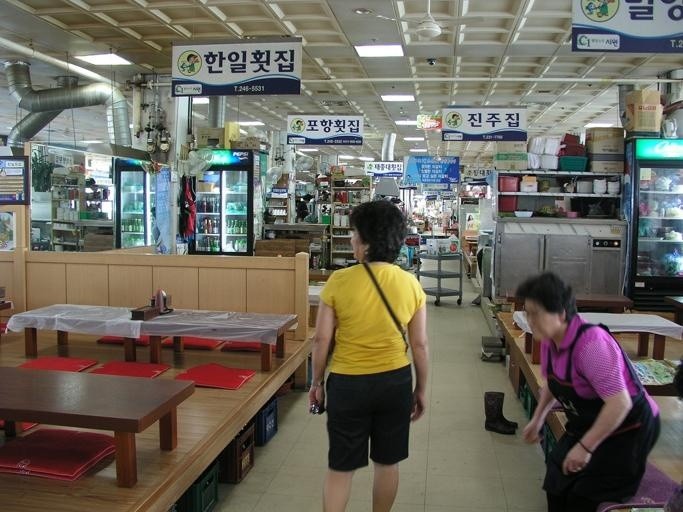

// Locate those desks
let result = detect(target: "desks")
[506,286,683,364]
[1,364,196,487]
[142,307,299,370]
[12,301,142,362]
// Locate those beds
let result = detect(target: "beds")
[495,312,683,512]
[0,244,320,512]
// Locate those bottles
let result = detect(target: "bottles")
[120,214,143,234]
[195,196,247,252]
[154,288,165,314]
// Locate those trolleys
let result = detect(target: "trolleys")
[415,248,465,307]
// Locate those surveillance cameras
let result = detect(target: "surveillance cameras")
[425,57,439,67]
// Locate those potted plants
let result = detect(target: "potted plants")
[31,149,65,203]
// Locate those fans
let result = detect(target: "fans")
[375,0,485,41]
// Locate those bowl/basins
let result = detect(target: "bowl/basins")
[56,205,78,221]
[593,179,620,195]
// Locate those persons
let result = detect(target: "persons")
[467,215,474,225]
[515,273,661,511]
[310,200,428,512]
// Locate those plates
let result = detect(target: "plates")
[0,212,14,249]
[513,211,534,217]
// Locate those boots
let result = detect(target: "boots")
[483,391,515,435]
[502,417,518,428]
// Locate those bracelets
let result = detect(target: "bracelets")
[577,438,593,456]
[311,380,324,388]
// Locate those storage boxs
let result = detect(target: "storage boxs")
[493,141,527,170]
[255,239,310,256]
[585,127,626,173]
[625,89,663,141]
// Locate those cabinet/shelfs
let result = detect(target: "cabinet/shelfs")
[262,170,372,267]
[491,169,628,305]
[51,173,87,252]
[116,159,156,248]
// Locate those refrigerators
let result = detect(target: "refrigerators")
[111,156,171,251]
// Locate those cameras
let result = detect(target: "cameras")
[311,404,320,415]
